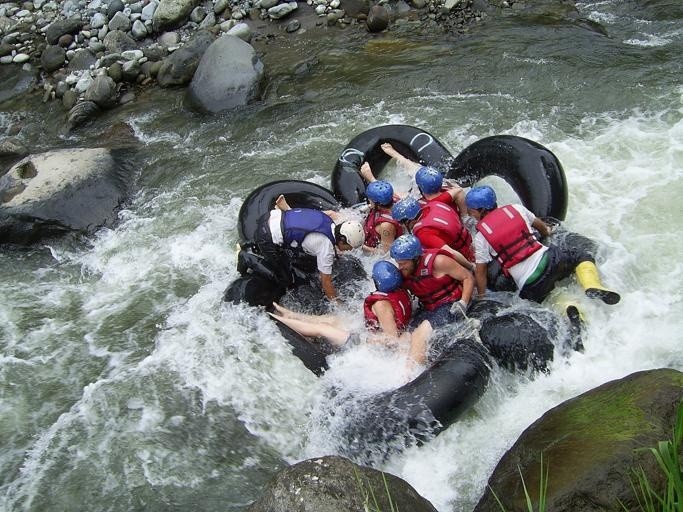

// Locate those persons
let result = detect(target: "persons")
[359,144,477,272]
[255,181,404,302]
[269,262,412,355]
[465,186,620,356]
[387,234,510,371]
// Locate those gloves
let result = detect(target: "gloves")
[450,301,468,318]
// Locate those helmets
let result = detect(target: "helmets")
[365,180,393,206]
[372,261,402,293]
[391,197,423,225]
[340,221,366,249]
[415,165,442,195]
[390,234,423,261]
[465,186,496,210]
